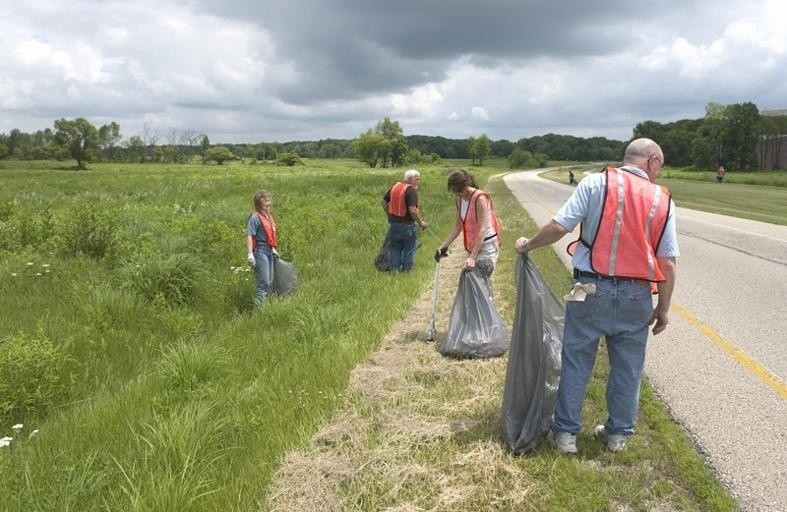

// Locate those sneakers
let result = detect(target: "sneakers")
[594,424,628,454]
[545,429,578,455]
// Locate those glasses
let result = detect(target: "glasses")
[647,154,665,174]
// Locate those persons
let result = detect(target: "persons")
[434,169,501,287]
[514,138,679,454]
[246,190,280,305]
[568,171,574,184]
[381,170,429,276]
[717,166,724,184]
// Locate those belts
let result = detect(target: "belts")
[579,270,639,281]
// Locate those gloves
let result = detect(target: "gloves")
[271,246,280,258]
[248,253,256,267]
